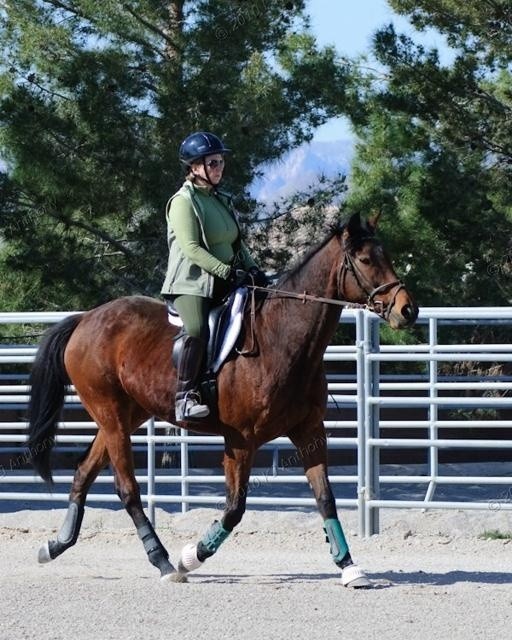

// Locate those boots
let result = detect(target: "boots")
[174,333,209,423]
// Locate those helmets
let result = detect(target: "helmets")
[179,131,230,162]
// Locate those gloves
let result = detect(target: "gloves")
[230,269,267,296]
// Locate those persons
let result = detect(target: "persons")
[160,132,269,422]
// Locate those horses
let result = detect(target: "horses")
[26,209,420,587]
